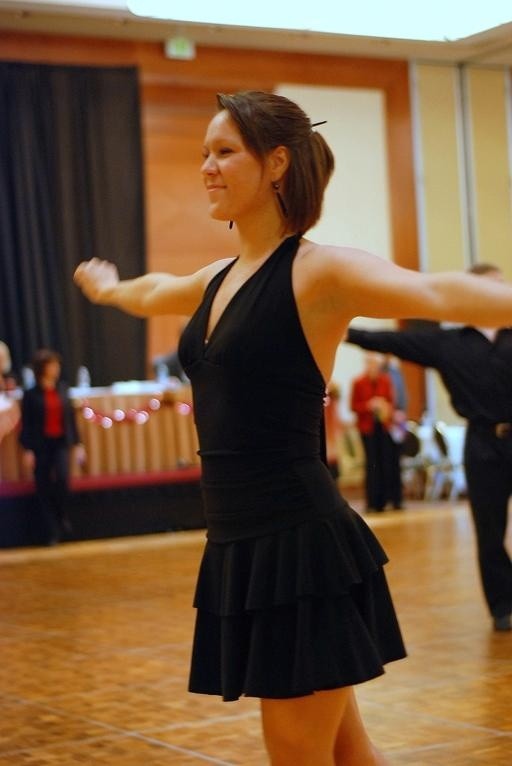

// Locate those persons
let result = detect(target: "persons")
[71,86,512,766]
[152,320,191,384]
[376,352,407,512]
[343,261,512,634]
[349,351,396,512]
[17,348,88,546]
